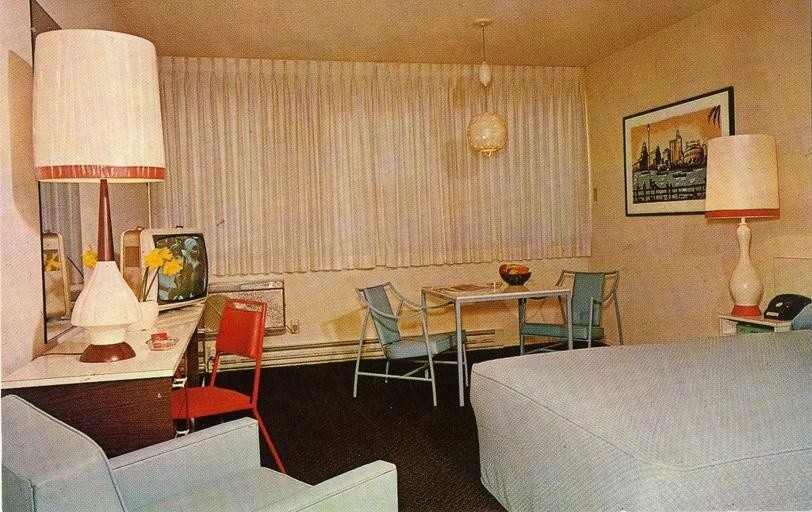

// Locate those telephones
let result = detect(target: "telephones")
[765,294,811,321]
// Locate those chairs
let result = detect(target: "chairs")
[518,268,625,355]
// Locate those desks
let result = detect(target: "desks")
[419,283,574,408]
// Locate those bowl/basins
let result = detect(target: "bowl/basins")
[500,272,531,285]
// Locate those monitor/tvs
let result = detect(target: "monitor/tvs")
[121,227,211,311]
[42,232,70,319]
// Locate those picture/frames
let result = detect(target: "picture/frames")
[621,85,735,217]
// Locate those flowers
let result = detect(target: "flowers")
[81,244,184,301]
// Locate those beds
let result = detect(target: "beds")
[470,307,812,511]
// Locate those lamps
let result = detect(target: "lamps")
[29,26,171,365]
[468,18,510,160]
[703,133,781,318]
